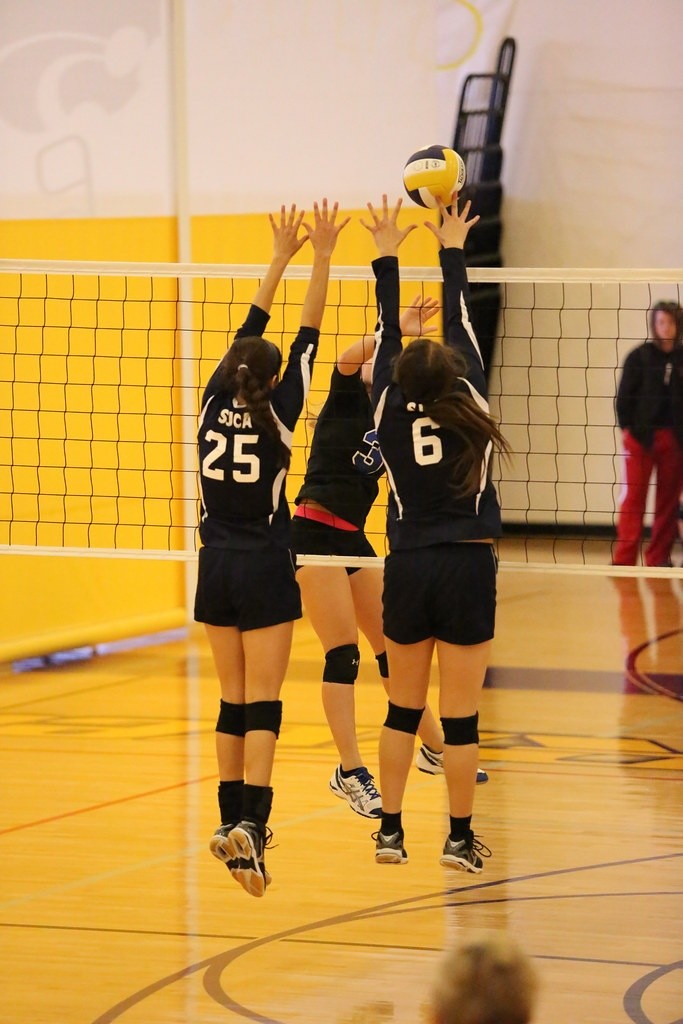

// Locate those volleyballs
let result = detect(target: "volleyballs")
[401,143,468,210]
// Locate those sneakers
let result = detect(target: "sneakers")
[328,763,383,819]
[371,830,408,864]
[209,823,271,887]
[415,743,489,785]
[440,830,492,874]
[228,819,265,897]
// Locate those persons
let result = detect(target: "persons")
[192,191,512,896]
[610,302,683,571]
